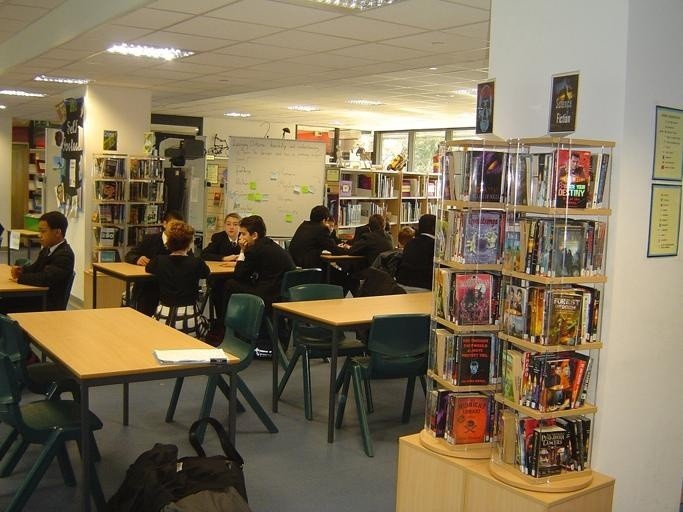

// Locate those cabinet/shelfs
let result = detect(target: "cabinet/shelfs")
[7,228,39,265]
[93,155,165,270]
[418,136,616,494]
[395,433,615,512]
[326,169,440,249]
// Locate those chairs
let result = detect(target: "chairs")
[335,313,429,457]
[0,352,104,512]
[279,284,375,420]
[0,314,102,463]
[164,293,279,444]
[156,263,212,335]
[267,268,321,370]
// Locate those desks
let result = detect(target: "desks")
[0,263,50,362]
[6,307,245,512]
[272,292,432,442]
[93,262,235,309]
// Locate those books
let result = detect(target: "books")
[93,158,165,270]
[155,348,230,365]
[432,328,593,413]
[436,268,601,346]
[426,388,591,478]
[437,209,606,277]
[336,175,423,227]
[442,149,610,210]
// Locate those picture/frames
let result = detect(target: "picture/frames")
[36,159,45,172]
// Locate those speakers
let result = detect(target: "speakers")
[183,140,204,161]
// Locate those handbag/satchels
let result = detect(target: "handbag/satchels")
[110,418,251,511]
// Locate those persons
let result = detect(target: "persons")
[223,215,297,318]
[11,211,75,364]
[373,214,436,291]
[559,152,585,178]
[145,220,214,339]
[346,214,394,296]
[203,214,241,320]
[288,205,349,298]
[125,208,187,307]
